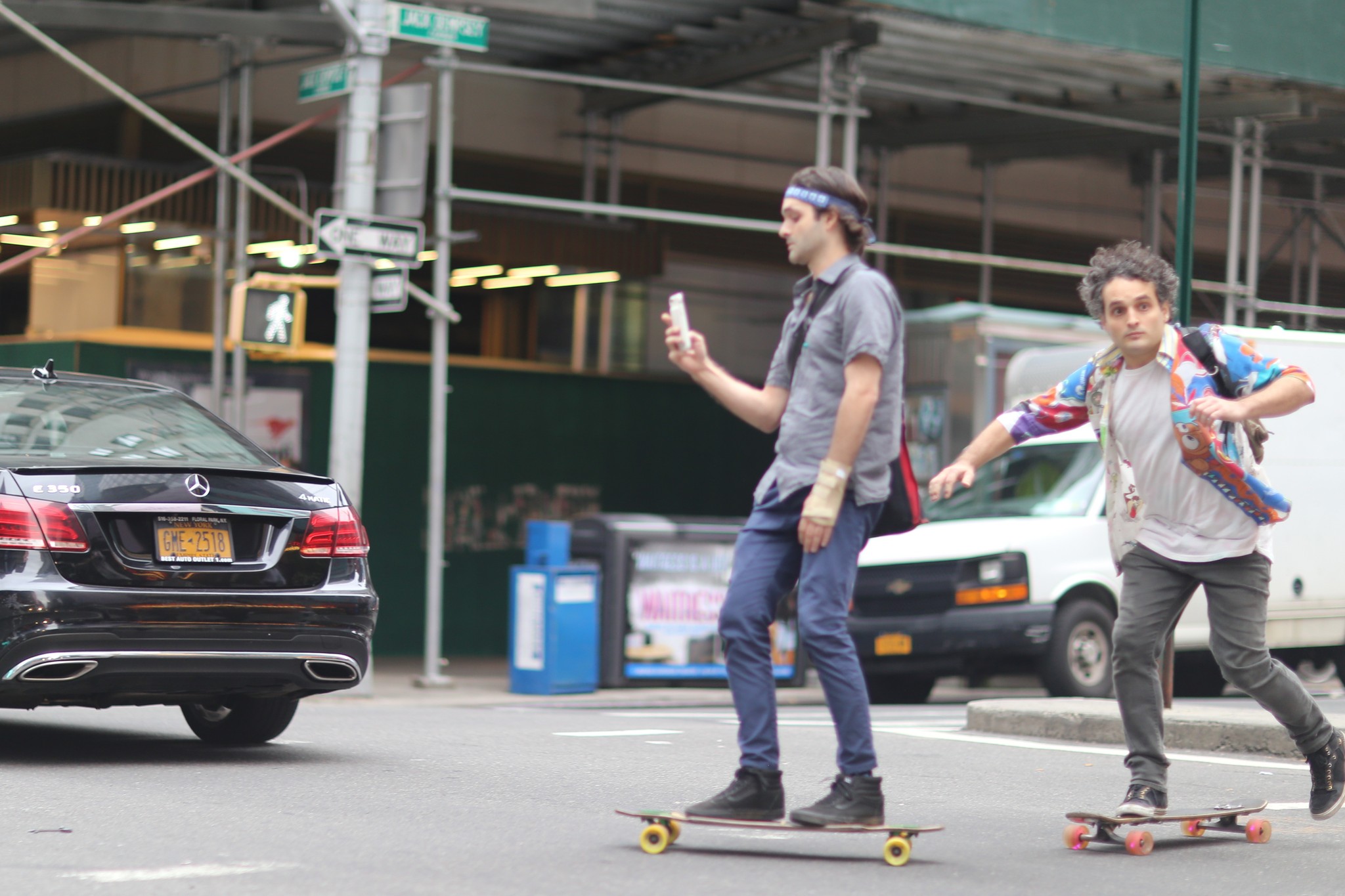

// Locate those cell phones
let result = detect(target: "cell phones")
[668,291,691,351]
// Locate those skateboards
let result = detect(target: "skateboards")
[611,808,946,865]
[1063,800,1278,855]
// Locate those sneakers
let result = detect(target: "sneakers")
[685,766,786,822]
[1117,782,1168,818]
[789,773,885,828]
[1302,728,1345,819]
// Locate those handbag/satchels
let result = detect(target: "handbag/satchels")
[867,437,923,537]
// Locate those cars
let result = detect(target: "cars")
[0,357,380,749]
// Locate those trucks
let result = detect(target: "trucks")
[842,322,1345,704]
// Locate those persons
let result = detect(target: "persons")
[660,163,905,831]
[929,242,1345,828]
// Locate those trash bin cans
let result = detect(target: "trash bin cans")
[506,520,602,695]
[572,511,807,688]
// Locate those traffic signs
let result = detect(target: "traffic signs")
[312,208,428,271]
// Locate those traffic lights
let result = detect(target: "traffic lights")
[229,278,307,350]
